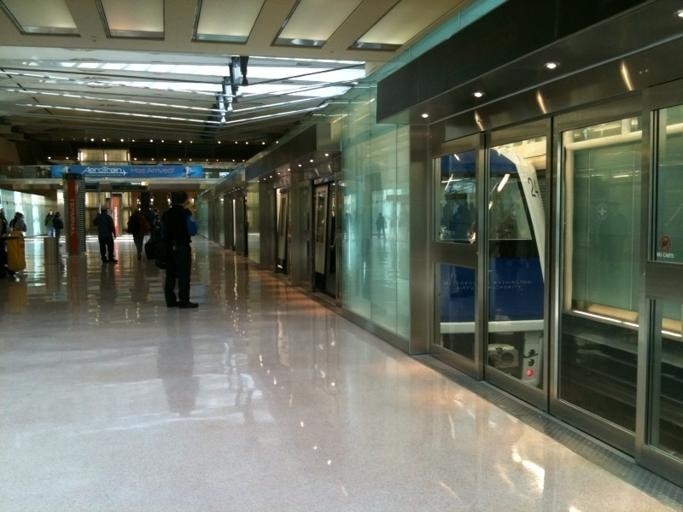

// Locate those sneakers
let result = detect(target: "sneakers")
[167,301,198,308]
[103,258,117,263]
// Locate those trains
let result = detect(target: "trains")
[275,143,559,387]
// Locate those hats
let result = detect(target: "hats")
[101,205,109,211]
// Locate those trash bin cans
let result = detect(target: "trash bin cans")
[44,237,58,265]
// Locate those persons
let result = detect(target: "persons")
[52,211,63,238]
[597,200,628,289]
[92,205,118,264]
[375,212,388,239]
[445,196,473,240]
[159,188,199,309]
[157,304,199,424]
[128,259,150,306]
[11,213,27,233]
[126,209,149,261]
[45,212,55,237]
[95,261,118,322]
[150,207,161,229]
